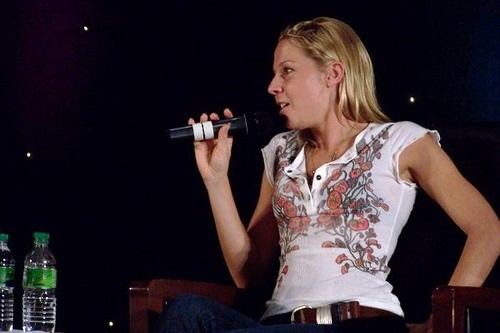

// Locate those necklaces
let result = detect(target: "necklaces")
[311,122,358,173]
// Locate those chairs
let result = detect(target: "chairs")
[294,285,500,333]
[129,278,238,333]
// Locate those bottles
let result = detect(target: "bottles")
[22,232,57,333]
[0,233,16,333]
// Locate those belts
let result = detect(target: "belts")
[261,301,394,326]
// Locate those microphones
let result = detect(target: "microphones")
[168,111,272,139]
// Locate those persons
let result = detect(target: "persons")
[153,17,500,333]
